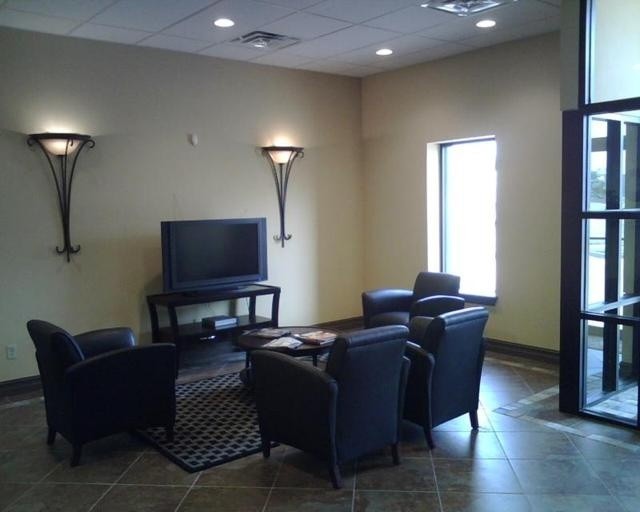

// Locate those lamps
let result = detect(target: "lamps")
[259,144,303,251]
[25,130,95,265]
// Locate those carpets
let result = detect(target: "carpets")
[135,354,309,475]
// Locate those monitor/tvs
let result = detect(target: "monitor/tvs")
[160,217,269,296]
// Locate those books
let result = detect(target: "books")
[261,336,304,351]
[292,330,338,346]
[202,315,238,328]
[243,327,291,339]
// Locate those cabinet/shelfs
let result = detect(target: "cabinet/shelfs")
[145,282,281,350]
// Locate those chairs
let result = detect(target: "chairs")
[244,323,410,491]
[361,270,469,334]
[25,318,179,469]
[396,306,490,448]
